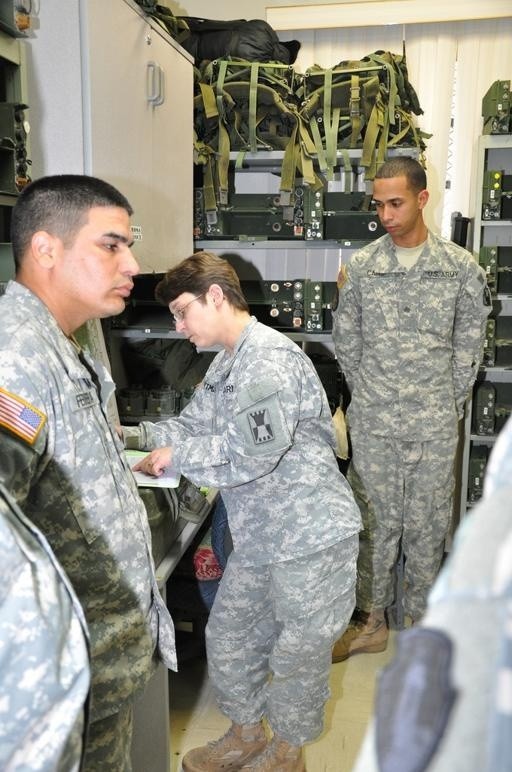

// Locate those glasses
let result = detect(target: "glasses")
[173,294,204,318]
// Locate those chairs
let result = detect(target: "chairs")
[159,493,240,666]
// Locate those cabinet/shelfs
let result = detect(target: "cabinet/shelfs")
[24,0,196,277]
[455,131,511,527]
[112,144,424,430]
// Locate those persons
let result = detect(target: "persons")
[331,157,492,663]
[0,175,178,772]
[113,252,364,772]
[352,417,512,772]
[0,486,92,772]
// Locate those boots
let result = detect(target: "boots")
[245,734,307,771]
[334,607,389,663]
[183,723,268,771]
